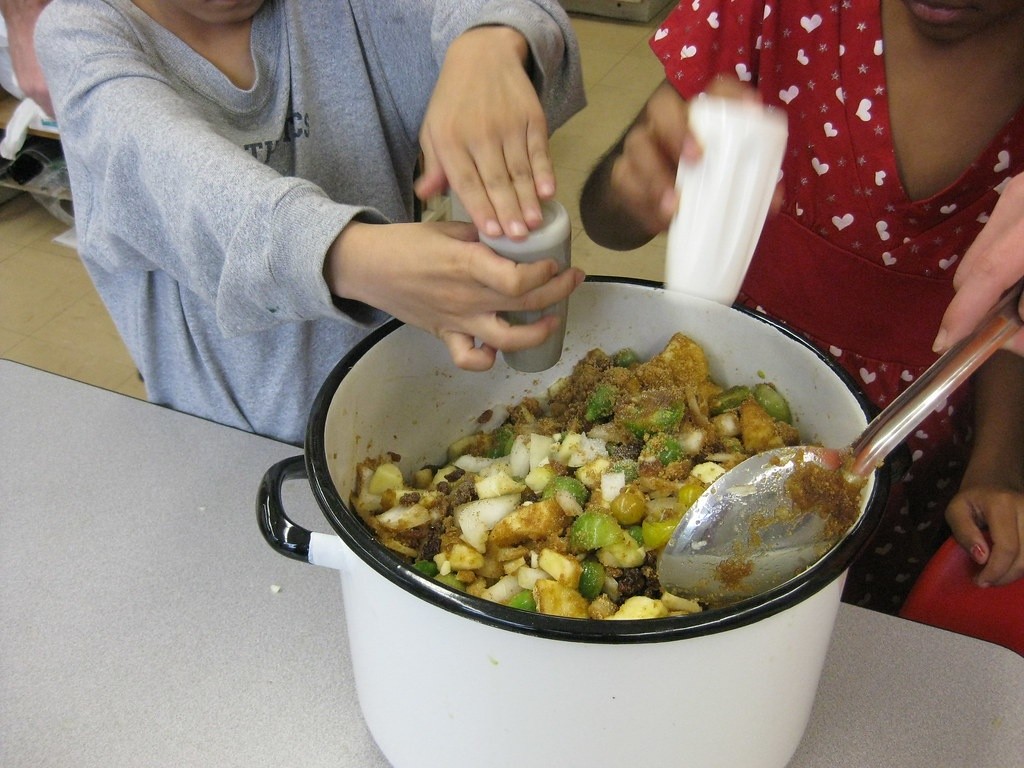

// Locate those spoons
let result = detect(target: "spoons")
[652,287,1024,618]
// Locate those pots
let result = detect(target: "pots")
[259,277,893,768]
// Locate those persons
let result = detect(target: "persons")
[931,169,1024,356]
[0,0,57,122]
[579,0,1024,617]
[33,0,589,451]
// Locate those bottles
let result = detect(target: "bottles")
[475,202,569,373]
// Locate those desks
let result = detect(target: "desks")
[0,357,1024,768]
